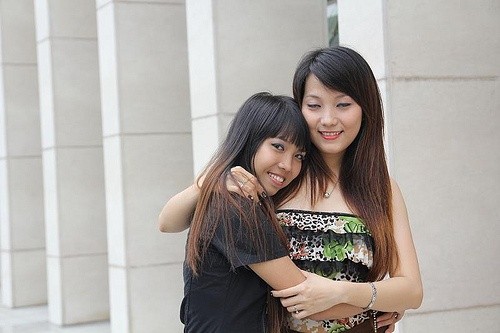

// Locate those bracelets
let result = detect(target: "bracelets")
[363,281,376,309]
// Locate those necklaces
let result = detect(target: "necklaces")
[315,174,339,198]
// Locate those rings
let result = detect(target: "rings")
[394,314,398,319]
[293,305,299,314]
[239,184,244,188]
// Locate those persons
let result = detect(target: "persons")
[158,45,423,333]
[180,92,405,333]
